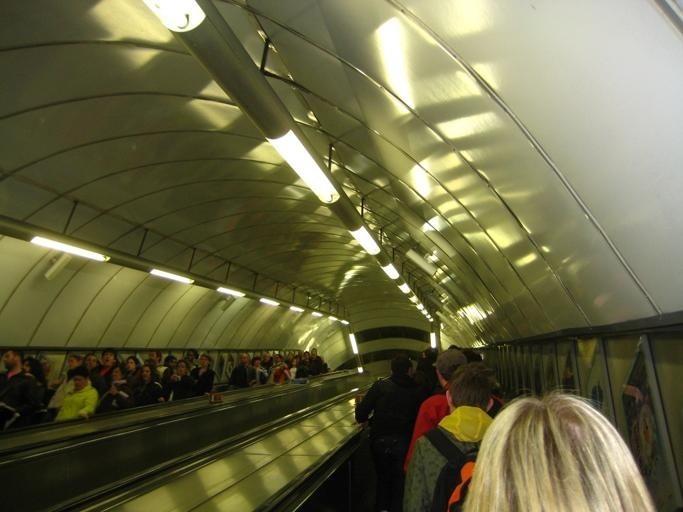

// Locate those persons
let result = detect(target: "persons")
[150,349,216,402]
[355,346,500,512]
[1,348,164,433]
[462,388,657,512]
[229,348,329,390]
